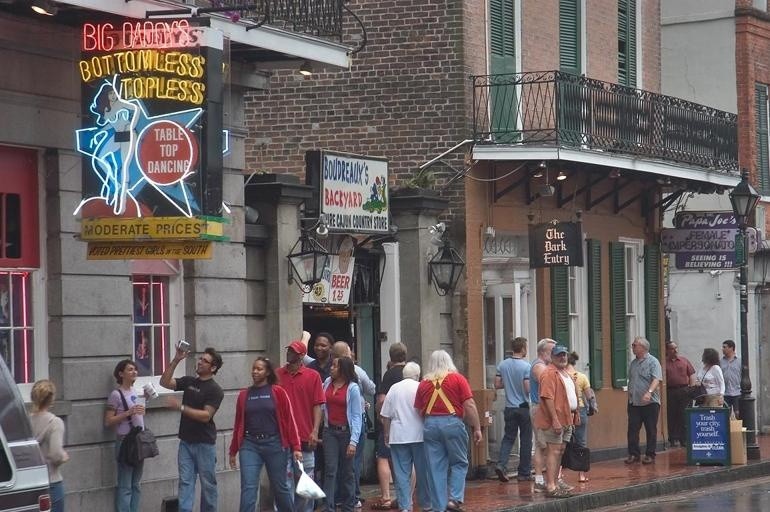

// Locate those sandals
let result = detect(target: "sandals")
[371,498,391,510]
[447,500,464,512]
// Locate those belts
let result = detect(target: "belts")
[246,432,276,439]
[329,426,348,431]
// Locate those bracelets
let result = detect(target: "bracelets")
[178,404,186,414]
[648,386,655,395]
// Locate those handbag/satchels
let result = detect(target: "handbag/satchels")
[562,443,590,471]
[689,384,706,399]
[118,426,159,466]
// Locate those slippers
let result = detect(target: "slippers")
[579,477,588,482]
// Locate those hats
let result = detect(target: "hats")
[552,345,568,355]
[285,341,306,354]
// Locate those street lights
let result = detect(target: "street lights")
[728,168,759,458]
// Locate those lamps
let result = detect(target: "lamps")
[300,61,314,76]
[608,167,621,178]
[31,0,58,16]
[532,160,567,196]
[285,217,329,293]
[656,174,665,184]
[428,224,466,297]
[666,176,672,184]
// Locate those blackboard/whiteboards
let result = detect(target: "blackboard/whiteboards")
[528,221,584,267]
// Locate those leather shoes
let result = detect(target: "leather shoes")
[624,456,639,464]
[642,455,655,464]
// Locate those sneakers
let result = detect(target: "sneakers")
[518,474,536,481]
[534,478,575,498]
[336,496,366,507]
[495,465,509,482]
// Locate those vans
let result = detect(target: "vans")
[1,351,53,512]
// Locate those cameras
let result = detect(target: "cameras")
[175,340,191,354]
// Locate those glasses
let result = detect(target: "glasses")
[200,357,209,363]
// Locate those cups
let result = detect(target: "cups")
[142,381,159,400]
[133,397,147,415]
[176,339,191,354]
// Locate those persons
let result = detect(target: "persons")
[494,336,597,497]
[158,340,224,512]
[104,360,152,512]
[664,340,743,450]
[270,329,433,512]
[92,83,140,215]
[226,356,303,512]
[412,347,483,512]
[25,378,69,512]
[624,336,665,465]
